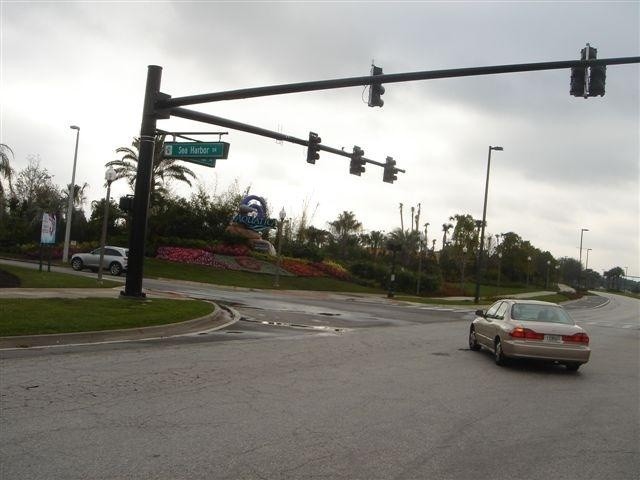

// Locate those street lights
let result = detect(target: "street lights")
[61,123,83,264]
[576,228,590,295]
[623,266,628,279]
[586,248,593,270]
[271,205,287,288]
[95,161,120,283]
[473,145,505,305]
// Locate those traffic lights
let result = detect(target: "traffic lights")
[382,155,400,184]
[348,142,367,177]
[366,63,387,108]
[306,131,323,166]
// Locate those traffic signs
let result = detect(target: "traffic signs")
[162,140,225,159]
[183,157,217,168]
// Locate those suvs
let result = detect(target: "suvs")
[68,243,129,275]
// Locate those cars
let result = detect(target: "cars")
[467,297,593,376]
